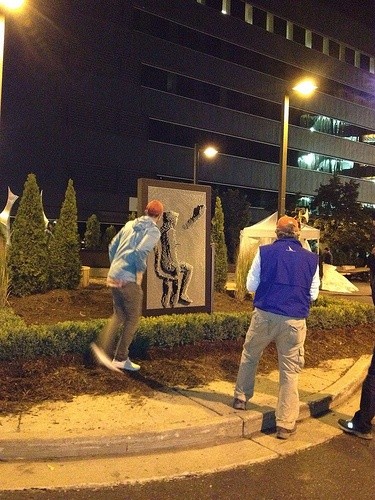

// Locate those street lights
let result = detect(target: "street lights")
[276,81,318,225]
[193,143,217,185]
[0,0,27,117]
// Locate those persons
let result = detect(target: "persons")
[337,247,375,439]
[324,247,333,265]
[234,216,320,439]
[91,200,163,374]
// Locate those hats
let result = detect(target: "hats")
[277,216,299,234]
[146,200,163,216]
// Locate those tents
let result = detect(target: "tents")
[236,211,320,302]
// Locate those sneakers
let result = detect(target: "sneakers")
[90,341,125,374]
[111,356,141,371]
[336,419,374,439]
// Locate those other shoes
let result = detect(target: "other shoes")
[233,398,247,410]
[276,426,297,438]
